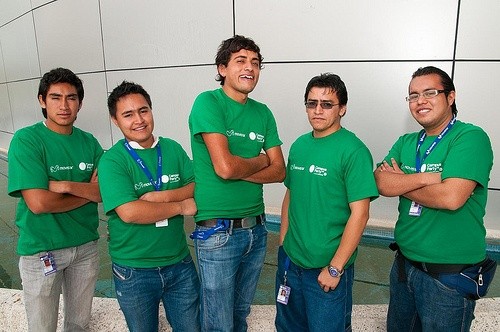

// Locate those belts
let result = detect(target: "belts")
[196,212,265,229]
[388,242,476,274]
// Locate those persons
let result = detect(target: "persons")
[274,74,380,332]
[279,290,286,301]
[194,35,286,332]
[374,66,493,332]
[97,82,197,332]
[7,68,104,332]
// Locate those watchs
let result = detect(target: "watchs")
[328,265,344,277]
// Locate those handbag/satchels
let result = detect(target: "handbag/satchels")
[432,255,497,300]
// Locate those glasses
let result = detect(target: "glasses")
[406,89,453,103]
[305,102,344,109]
